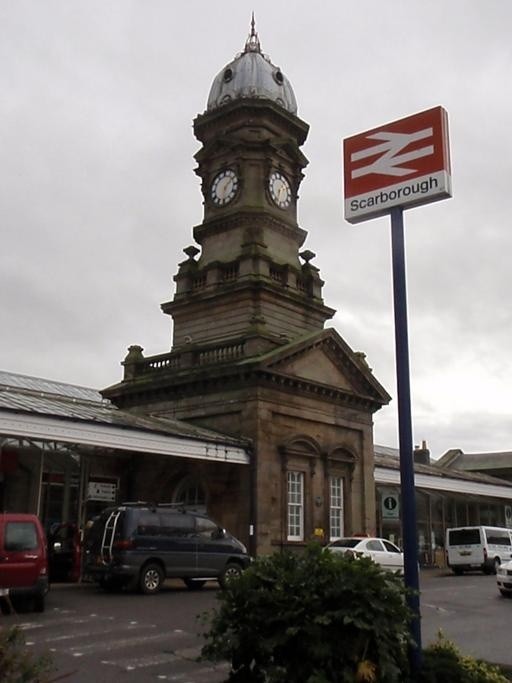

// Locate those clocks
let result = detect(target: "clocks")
[266,166,293,211]
[208,166,241,208]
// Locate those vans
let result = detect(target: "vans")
[445,524,512,574]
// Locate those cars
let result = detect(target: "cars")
[322,533,422,576]
[494,558,512,597]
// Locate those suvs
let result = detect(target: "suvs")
[83,500,253,594]
[0,510,52,613]
